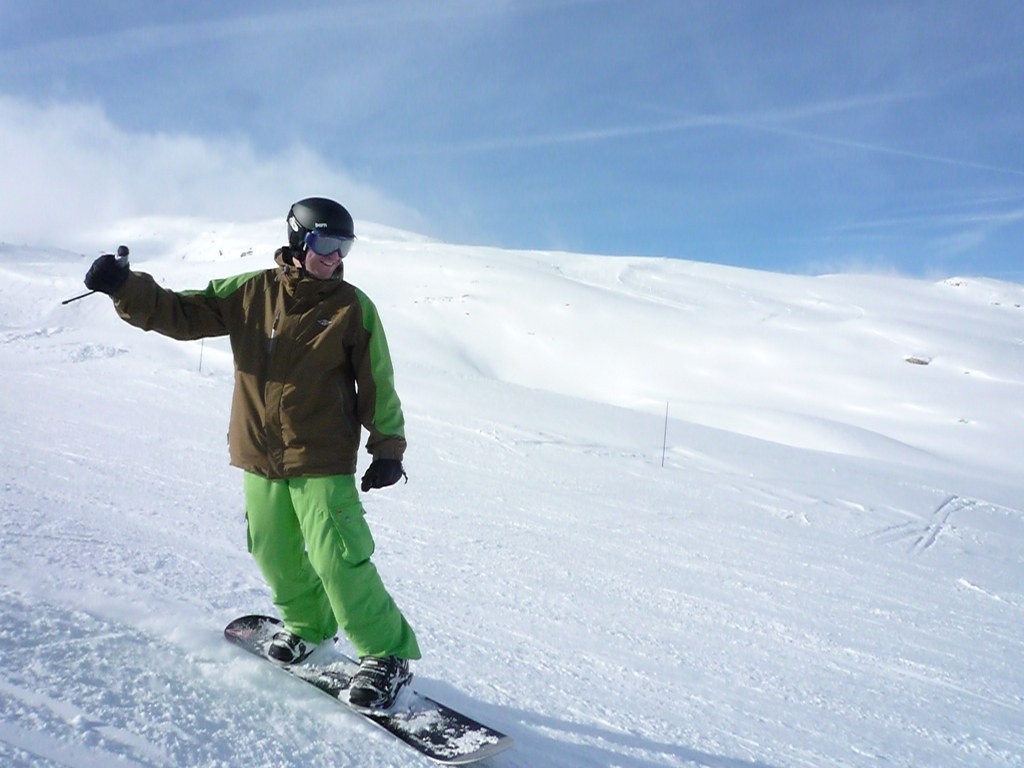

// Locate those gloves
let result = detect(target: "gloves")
[361,457,403,491]
[83,246,130,294]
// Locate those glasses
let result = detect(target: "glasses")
[305,229,355,258]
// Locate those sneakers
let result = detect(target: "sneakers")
[348,655,412,710]
[266,626,338,665]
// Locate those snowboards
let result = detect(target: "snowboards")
[225,614,515,766]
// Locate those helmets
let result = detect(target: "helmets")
[286,198,355,258]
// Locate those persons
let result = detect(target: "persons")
[83,197,422,711]
